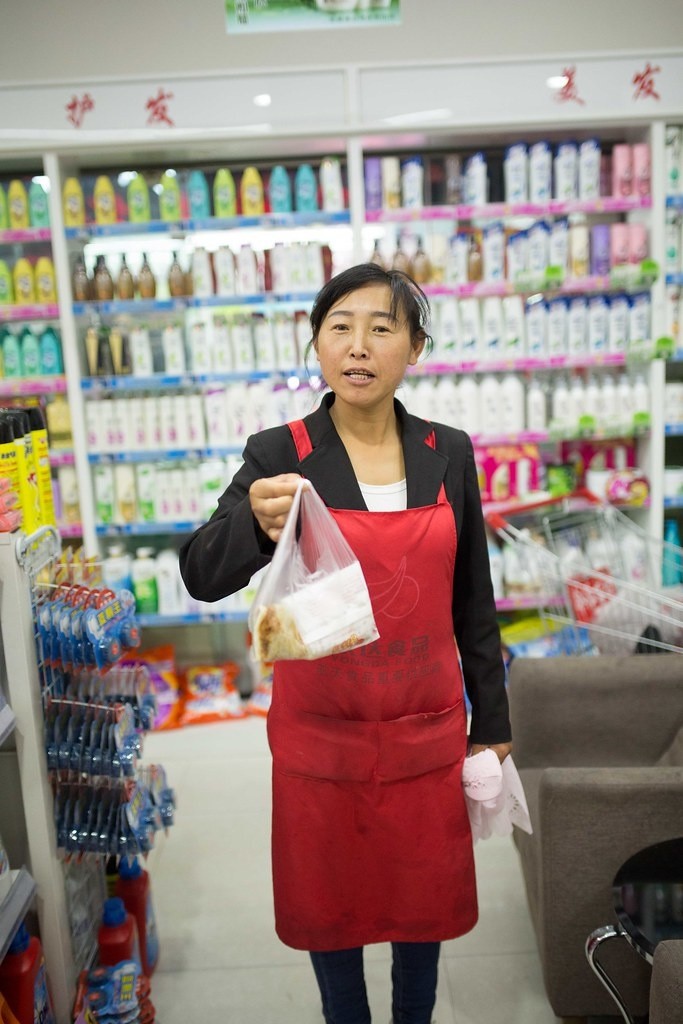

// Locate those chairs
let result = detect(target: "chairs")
[503,653,683,1024]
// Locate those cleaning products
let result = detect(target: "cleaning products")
[2,849,161,1022]
[39,619,606,731]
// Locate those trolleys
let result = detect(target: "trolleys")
[481,487,682,656]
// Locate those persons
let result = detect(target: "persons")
[179,263,513,1024]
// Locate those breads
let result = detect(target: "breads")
[256,605,366,661]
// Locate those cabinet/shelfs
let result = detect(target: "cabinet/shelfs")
[0,111,683,1024]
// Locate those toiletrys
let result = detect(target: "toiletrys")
[2,134,683,617]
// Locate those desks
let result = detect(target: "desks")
[584,837,683,1024]
